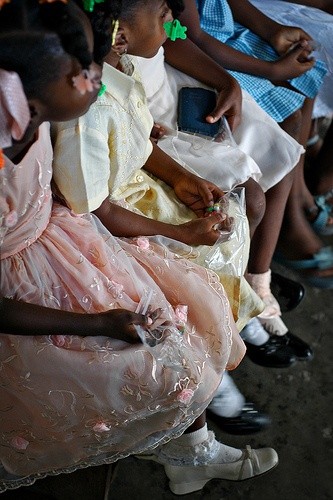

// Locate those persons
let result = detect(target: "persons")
[253,2,333,194]
[0,0,284,493]
[163,0,332,288]
[122,22,316,363]
[48,0,300,431]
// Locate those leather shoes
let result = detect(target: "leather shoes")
[271,271,305,312]
[207,396,275,436]
[240,330,298,369]
[264,327,313,361]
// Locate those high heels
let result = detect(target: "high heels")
[165,444,280,495]
[134,452,165,465]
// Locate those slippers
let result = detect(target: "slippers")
[273,238,333,289]
[313,193,332,236]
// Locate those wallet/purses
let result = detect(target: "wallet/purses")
[177,85,223,141]
